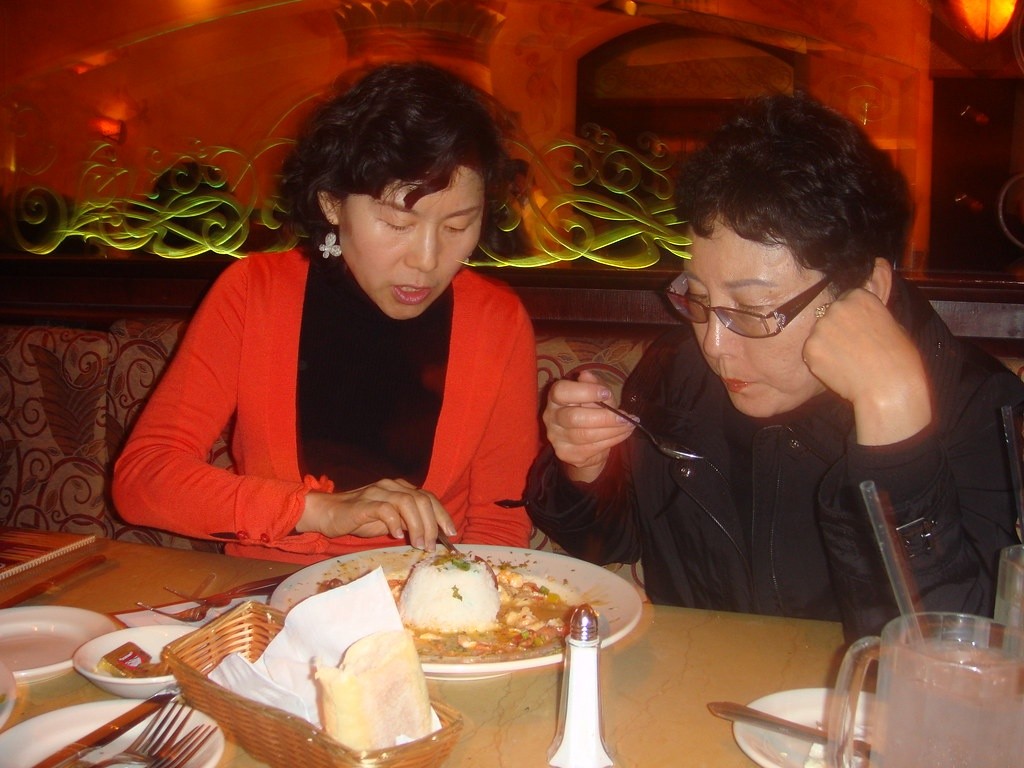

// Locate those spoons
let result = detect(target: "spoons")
[596,400,704,461]
[437,525,498,590]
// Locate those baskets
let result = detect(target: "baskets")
[160,601,463,768]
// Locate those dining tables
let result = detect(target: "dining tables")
[0,537,1024,768]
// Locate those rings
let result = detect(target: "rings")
[815,301,832,318]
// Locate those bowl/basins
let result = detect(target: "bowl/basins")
[0,660,16,729]
[74,625,201,699]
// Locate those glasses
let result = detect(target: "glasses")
[666,275,835,338]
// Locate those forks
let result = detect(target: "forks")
[164,581,277,607]
[136,586,250,622]
[90,701,196,768]
[145,725,217,768]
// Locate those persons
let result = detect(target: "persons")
[520,88,1024,677]
[110,60,540,562]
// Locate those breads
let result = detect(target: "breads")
[311,628,433,756]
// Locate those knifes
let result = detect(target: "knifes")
[31,683,183,768]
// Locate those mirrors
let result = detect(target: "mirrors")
[0,0,1023,359]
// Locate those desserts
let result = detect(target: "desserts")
[395,551,501,633]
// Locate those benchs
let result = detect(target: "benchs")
[0,319,1024,604]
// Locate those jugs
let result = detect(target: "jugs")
[827,612,1024,768]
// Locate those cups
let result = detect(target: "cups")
[994,545,1024,629]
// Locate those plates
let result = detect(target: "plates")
[0,606,117,686]
[271,544,641,680]
[732,687,875,768]
